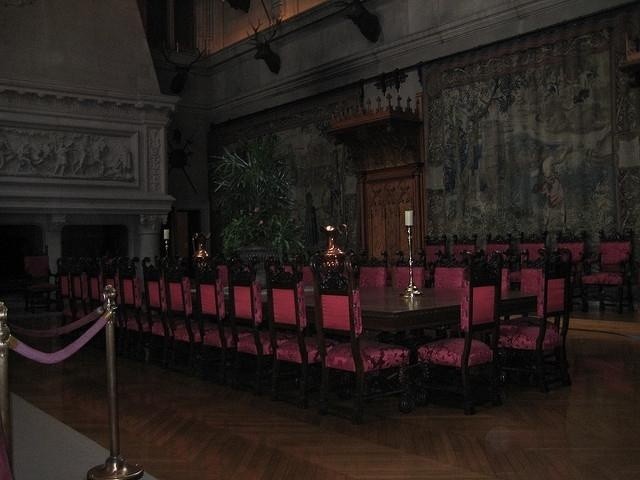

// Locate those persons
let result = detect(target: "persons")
[0,136,132,177]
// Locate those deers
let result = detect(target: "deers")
[154,40,208,94]
[243,15,281,74]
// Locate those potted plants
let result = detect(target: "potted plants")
[206,133,315,268]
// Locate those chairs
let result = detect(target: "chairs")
[556,238,592,314]
[581,237,635,316]
[24,229,574,427]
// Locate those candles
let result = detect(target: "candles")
[404,210,414,226]
